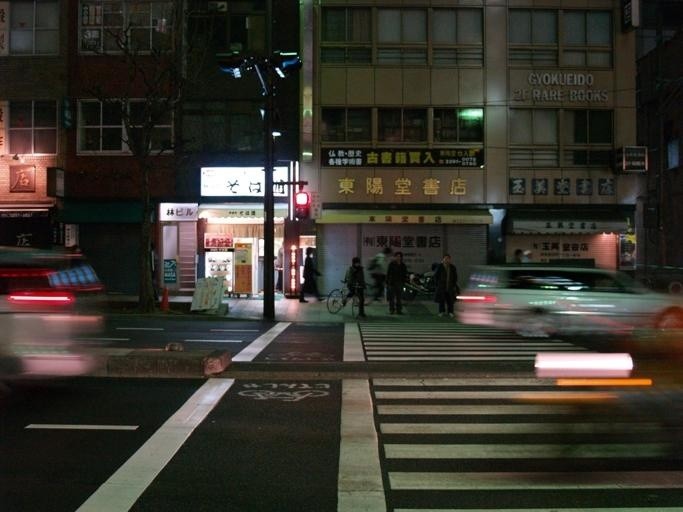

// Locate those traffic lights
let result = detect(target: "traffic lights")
[215,51,244,80]
[272,50,304,79]
[294,192,309,206]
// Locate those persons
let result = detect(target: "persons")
[299,247,325,303]
[341,257,367,318]
[276,242,284,293]
[367,247,408,315]
[511,248,534,264]
[431,254,457,319]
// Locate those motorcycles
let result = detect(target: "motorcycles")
[400,271,460,301]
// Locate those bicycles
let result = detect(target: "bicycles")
[327,280,369,320]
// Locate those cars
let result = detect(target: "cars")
[0,246,105,412]
[453,265,683,338]
